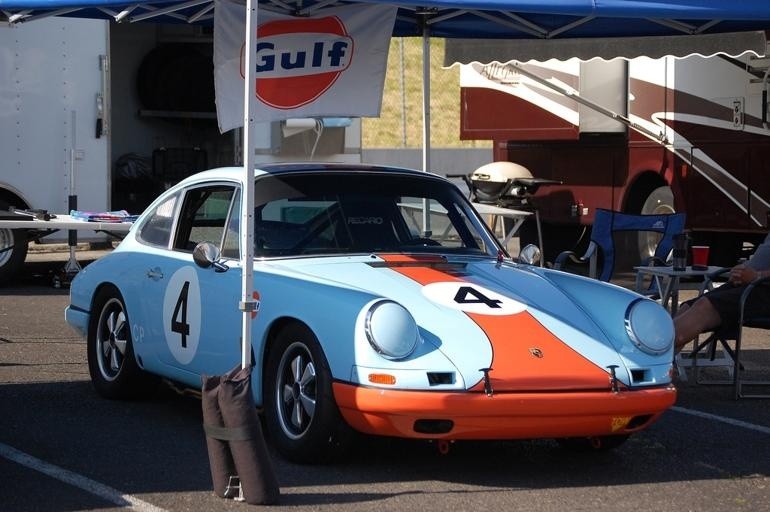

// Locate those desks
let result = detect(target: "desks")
[396,202,535,253]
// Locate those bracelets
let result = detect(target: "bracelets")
[757,270,762,280]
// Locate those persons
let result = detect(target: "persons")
[674,230,770,358]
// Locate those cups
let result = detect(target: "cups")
[672,233,689,271]
[691,245,710,270]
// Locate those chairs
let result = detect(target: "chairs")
[333,206,400,253]
[687,266,770,401]
[556,208,686,302]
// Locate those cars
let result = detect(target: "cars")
[64,162,679,461]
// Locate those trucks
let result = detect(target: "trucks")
[0,15,247,290]
[461,44,770,282]
